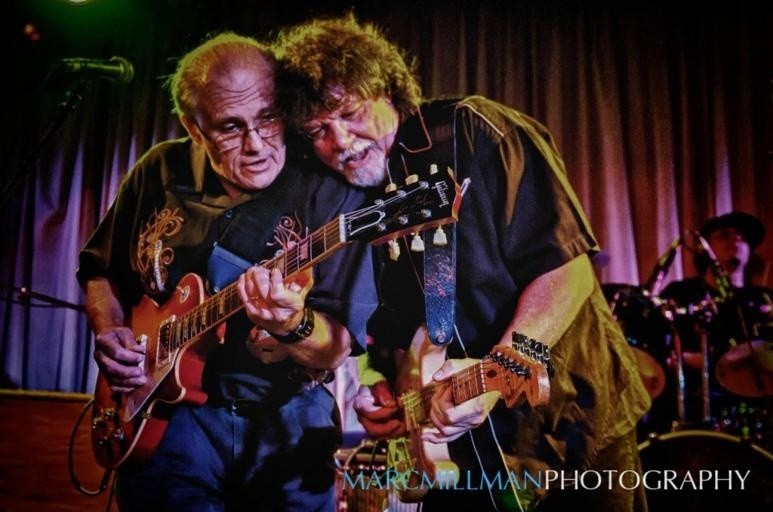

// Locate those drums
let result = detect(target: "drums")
[602,282,671,403]
[709,286,772,397]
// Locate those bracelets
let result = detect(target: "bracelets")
[268,307,314,345]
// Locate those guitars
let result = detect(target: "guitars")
[91,163,463,470]
[384,322,555,500]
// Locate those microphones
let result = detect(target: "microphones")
[62,54,135,85]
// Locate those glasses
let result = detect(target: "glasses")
[193,112,288,152]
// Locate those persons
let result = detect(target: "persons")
[272,10,654,512]
[75,33,379,511]
[659,209,773,428]
